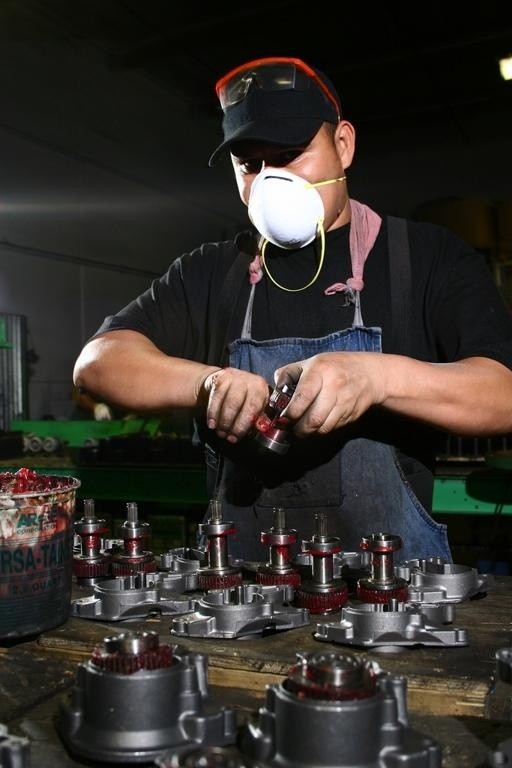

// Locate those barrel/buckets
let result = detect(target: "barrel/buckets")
[0,472,82,638]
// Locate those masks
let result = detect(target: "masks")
[248,168,346,292]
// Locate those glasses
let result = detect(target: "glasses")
[214,57,341,124]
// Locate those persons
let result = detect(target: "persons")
[73,382,138,422]
[72,24,511,577]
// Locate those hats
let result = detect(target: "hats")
[207,48,343,165]
